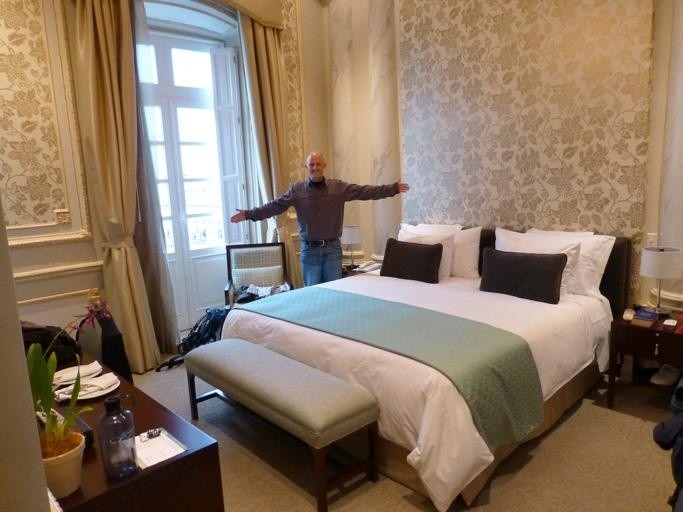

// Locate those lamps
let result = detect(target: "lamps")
[340,225,365,270]
[638,247,683,318]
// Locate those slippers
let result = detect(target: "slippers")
[650,364,680,386]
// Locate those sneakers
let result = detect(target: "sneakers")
[669,377,683,413]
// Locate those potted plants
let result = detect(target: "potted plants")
[25,343,87,500]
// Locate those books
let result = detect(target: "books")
[632,310,656,321]
[630,311,659,329]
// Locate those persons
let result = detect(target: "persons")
[228,150,411,290]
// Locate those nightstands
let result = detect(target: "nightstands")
[607,309,683,409]
[342,268,365,278]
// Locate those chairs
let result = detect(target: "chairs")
[223,242,294,307]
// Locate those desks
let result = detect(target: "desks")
[48,345,224,512]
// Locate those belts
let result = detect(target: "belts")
[303,237,338,247]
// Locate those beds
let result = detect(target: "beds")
[220,237,629,512]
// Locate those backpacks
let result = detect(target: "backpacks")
[182,308,229,354]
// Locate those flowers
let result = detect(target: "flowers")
[41,289,112,360]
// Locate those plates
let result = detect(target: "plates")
[49,366,121,400]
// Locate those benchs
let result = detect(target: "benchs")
[185,337,380,511]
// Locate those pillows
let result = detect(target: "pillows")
[234,266,283,294]
[380,222,617,304]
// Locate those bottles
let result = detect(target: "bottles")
[96,396,135,480]
